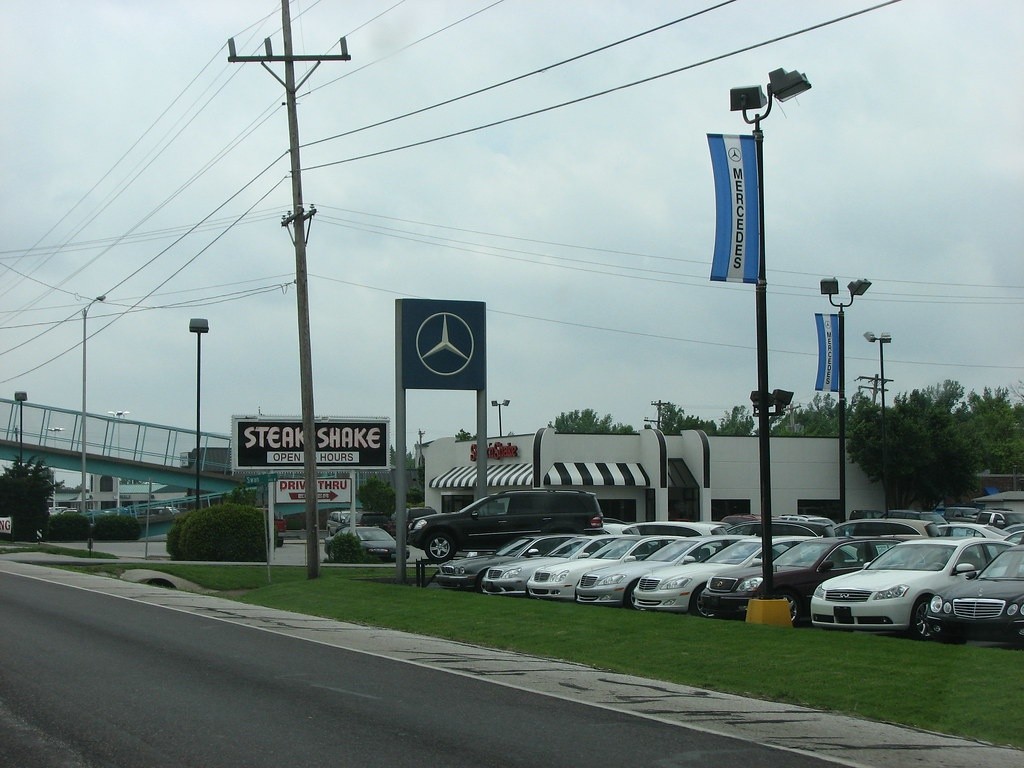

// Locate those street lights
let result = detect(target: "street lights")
[15,391,27,469]
[864,331,893,518]
[490,399,510,438]
[45,427,64,512]
[109,409,130,517]
[80,294,106,517]
[724,64,813,600]
[188,318,209,512]
[818,276,873,527]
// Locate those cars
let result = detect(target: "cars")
[699,507,1024,547]
[699,534,922,622]
[326,511,358,535]
[631,520,729,538]
[527,534,685,601]
[480,534,627,596]
[601,522,642,539]
[436,536,548,594]
[632,535,819,618]
[575,534,759,610]
[925,543,1024,648]
[813,536,1021,641]
[323,526,411,561]
[49,504,185,523]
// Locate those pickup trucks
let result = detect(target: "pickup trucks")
[256,509,285,547]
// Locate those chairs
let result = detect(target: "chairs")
[830,549,853,567]
[699,548,709,560]
[959,551,981,572]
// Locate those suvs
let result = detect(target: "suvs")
[338,512,390,535]
[406,482,604,563]
[392,505,436,528]
